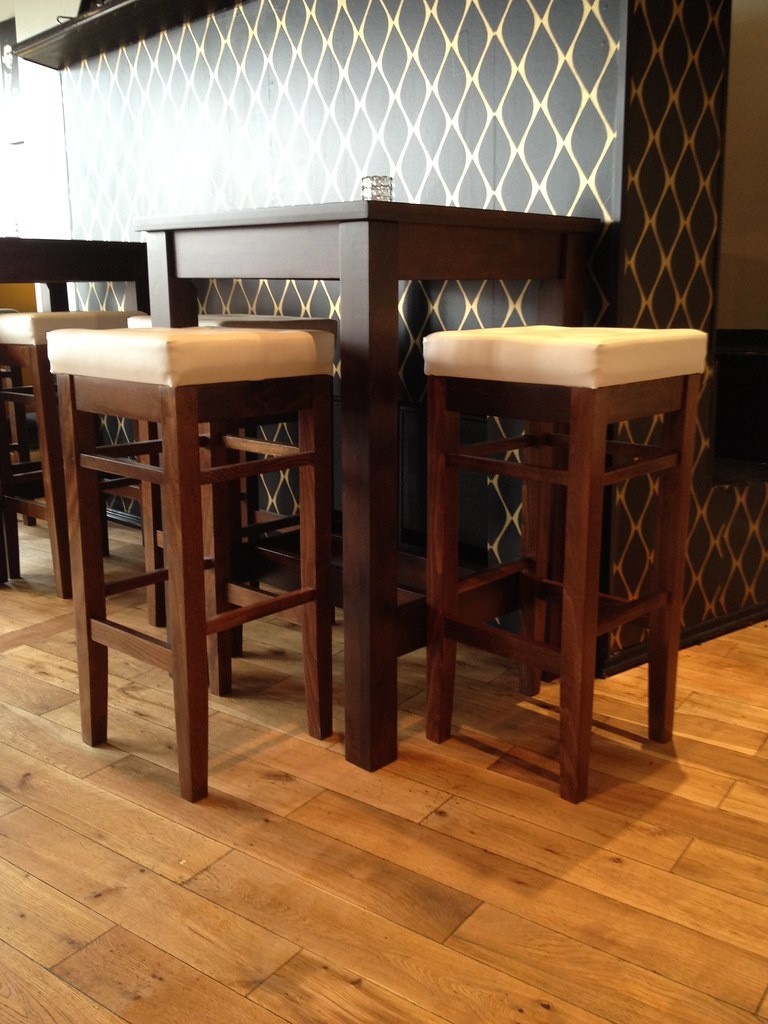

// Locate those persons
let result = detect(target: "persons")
[1,39,17,91]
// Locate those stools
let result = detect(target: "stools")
[421,325,708,807]
[0,311,337,806]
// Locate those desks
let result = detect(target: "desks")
[133,200,604,774]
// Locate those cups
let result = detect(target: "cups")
[361,176,392,201]
